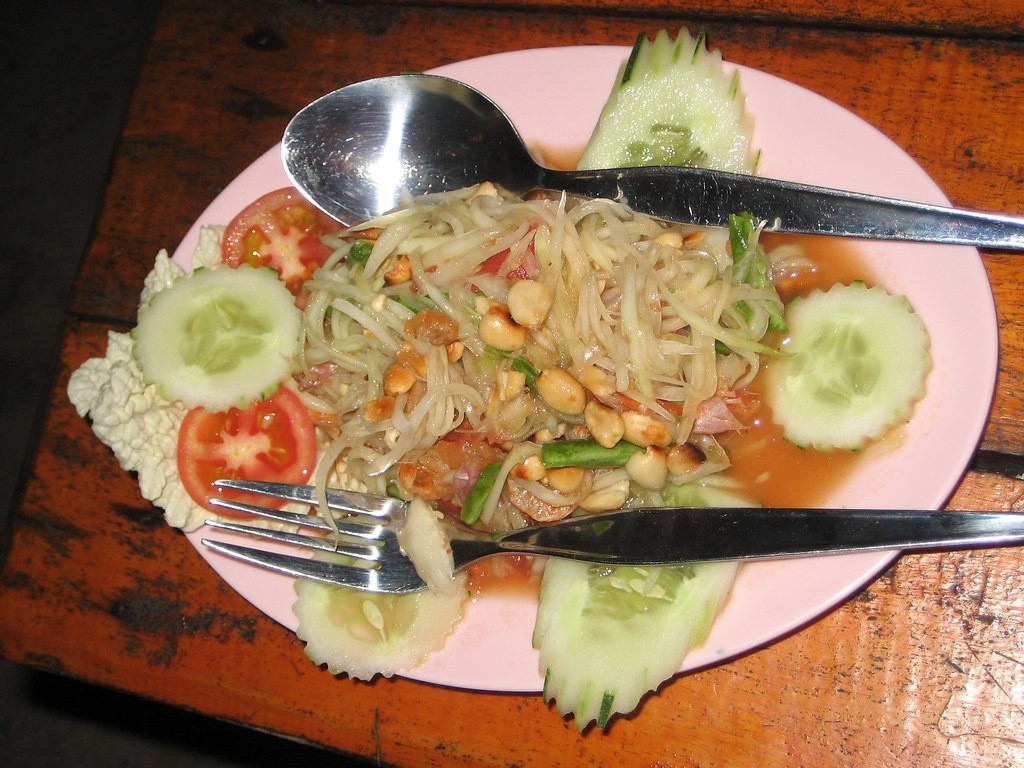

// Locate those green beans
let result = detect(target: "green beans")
[538,438,640,468]
[460,461,502,524]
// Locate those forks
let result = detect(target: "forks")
[201,478,1024,593]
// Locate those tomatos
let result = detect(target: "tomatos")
[221,186,344,307]
[176,384,317,523]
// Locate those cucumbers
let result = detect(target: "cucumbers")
[768,284,934,450]
[134,262,303,413]
[578,28,759,175]
[533,486,766,734]
[292,512,470,680]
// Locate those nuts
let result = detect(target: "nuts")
[303,218,706,522]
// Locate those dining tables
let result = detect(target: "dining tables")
[0,0,1024,768]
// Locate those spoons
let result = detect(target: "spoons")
[281,72,1024,251]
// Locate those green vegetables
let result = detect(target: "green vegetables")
[347,240,372,270]
[703,212,789,350]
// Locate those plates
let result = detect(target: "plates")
[161,42,992,695]
